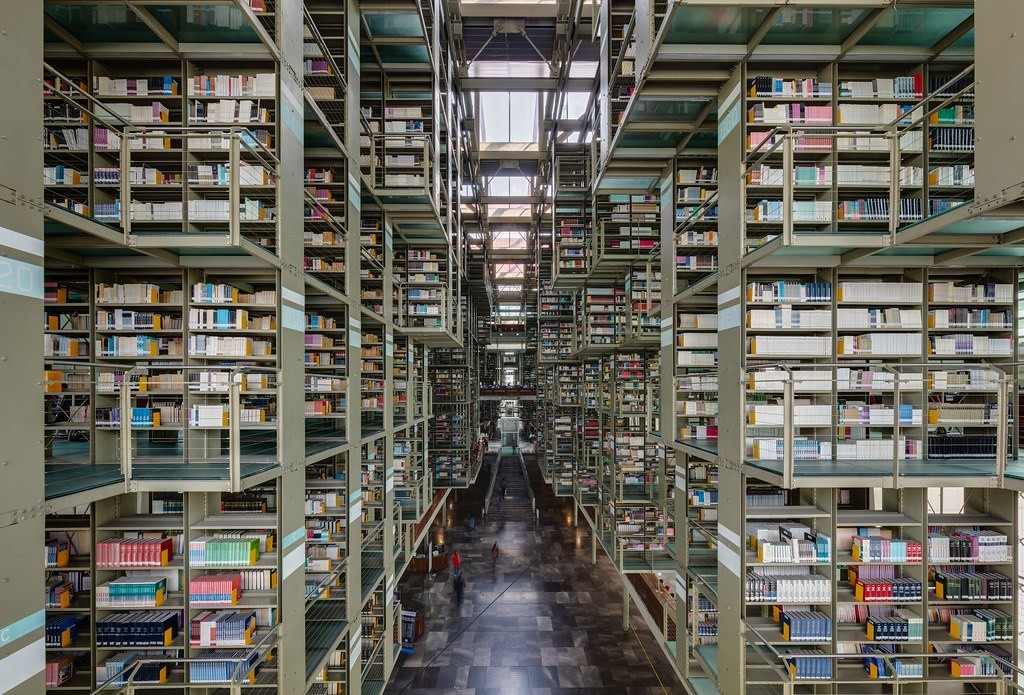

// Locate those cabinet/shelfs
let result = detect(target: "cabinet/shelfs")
[43,1,499,695]
[534,0,1024,695]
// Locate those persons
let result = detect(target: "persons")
[474,444,482,461]
[511,441,516,455]
[432,543,439,552]
[528,431,536,443]
[499,478,507,500]
[484,438,488,451]
[452,550,462,577]
[454,576,466,607]
[496,497,503,512]
[487,463,494,475]
[537,428,543,448]
[491,544,499,568]
